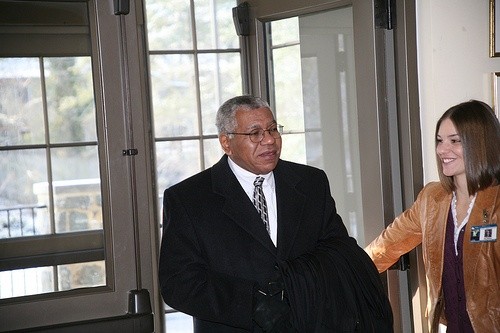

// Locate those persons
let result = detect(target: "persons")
[361,99,500,333]
[157,94,351,333]
[485,229,491,237]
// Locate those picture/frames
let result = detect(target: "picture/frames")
[488,0,500,58]
[491,71,500,122]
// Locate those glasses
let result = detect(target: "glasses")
[227,124,283,143]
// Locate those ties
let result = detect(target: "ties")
[253,176,270,236]
